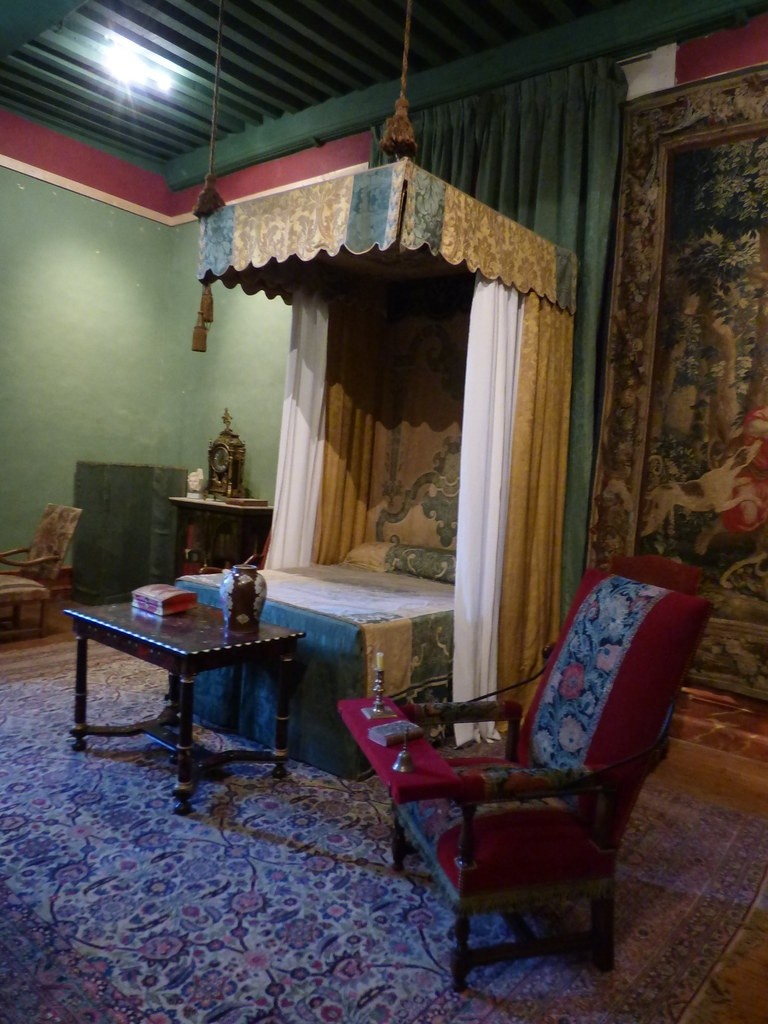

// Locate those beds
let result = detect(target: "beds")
[171,156,579,782]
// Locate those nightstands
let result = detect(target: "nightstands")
[167,496,274,578]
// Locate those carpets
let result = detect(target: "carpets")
[0,639,768,1024]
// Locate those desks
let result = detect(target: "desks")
[62,602,306,815]
[337,696,462,805]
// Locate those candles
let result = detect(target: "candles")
[376,650,384,671]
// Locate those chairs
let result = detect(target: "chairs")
[0,503,83,641]
[336,564,712,994]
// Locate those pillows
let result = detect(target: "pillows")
[343,541,457,584]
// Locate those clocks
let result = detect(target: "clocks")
[208,409,248,497]
